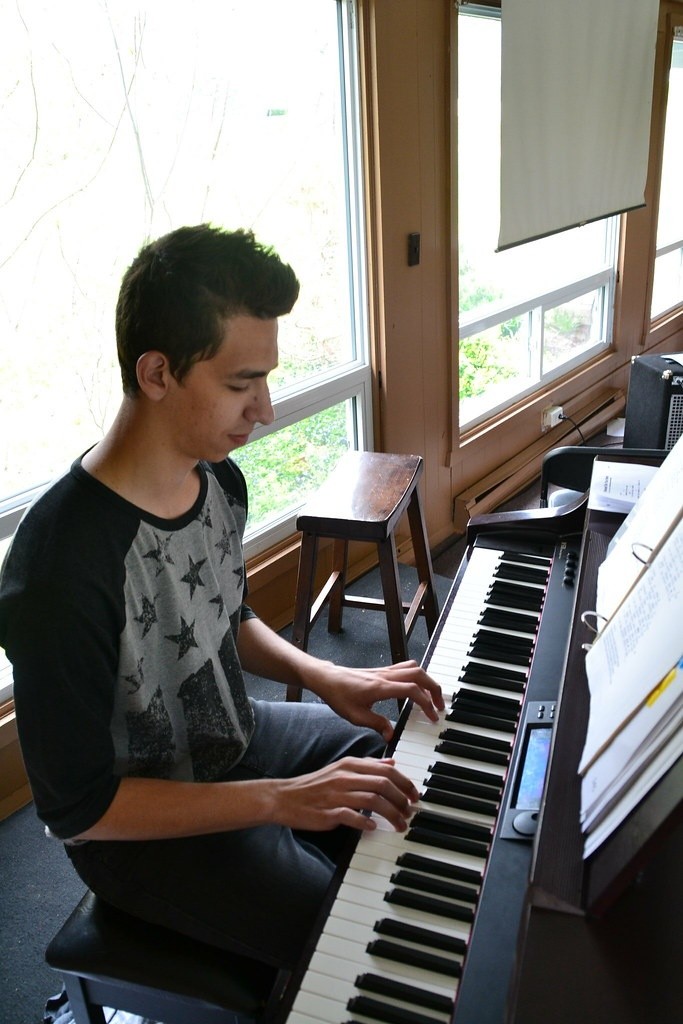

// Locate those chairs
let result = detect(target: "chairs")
[538,446,671,508]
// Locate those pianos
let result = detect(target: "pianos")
[258,444,683,1024]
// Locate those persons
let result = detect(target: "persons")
[0,223,449,1023]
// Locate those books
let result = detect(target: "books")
[578,430,682,865]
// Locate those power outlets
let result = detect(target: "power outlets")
[544,405,565,427]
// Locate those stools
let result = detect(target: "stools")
[44,888,270,1024]
[286,450,439,714]
